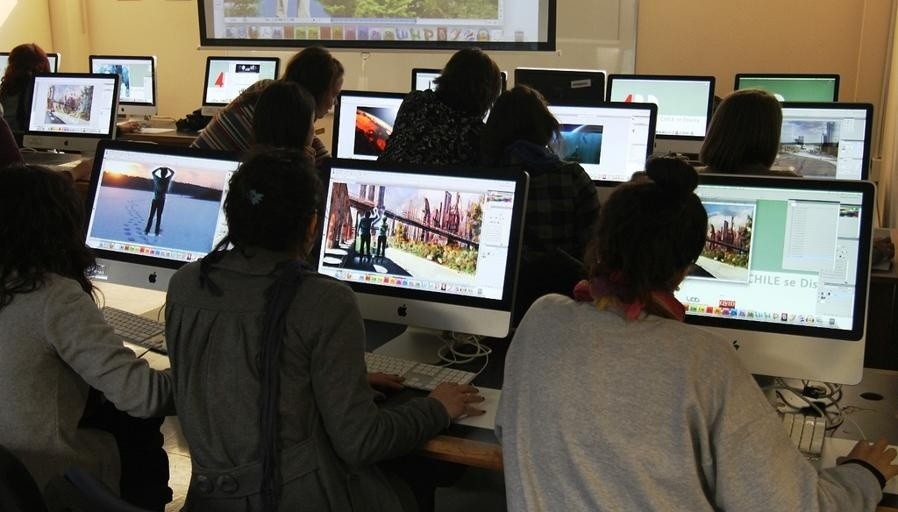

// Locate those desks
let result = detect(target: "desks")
[19,153,91,215]
[116,115,204,156]
[78,258,897,511]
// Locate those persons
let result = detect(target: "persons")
[143,167,175,235]
[379,45,500,167]
[696,90,895,267]
[374,212,390,259]
[164,156,485,512]
[0,43,51,136]
[0,165,174,511]
[457,86,599,284]
[250,78,317,153]
[357,208,376,257]
[189,44,343,158]
[496,153,898,511]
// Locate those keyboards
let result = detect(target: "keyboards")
[102,302,176,356]
[785,410,826,457]
[365,349,477,402]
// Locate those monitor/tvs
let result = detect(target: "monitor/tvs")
[332,89,409,160]
[19,72,121,148]
[313,155,531,362]
[733,73,843,103]
[200,54,280,115]
[409,63,506,96]
[545,98,659,186]
[772,102,875,178]
[677,171,878,385]
[518,64,604,100]
[84,52,156,119]
[77,138,246,290]
[0,49,58,77]
[605,74,714,158]
